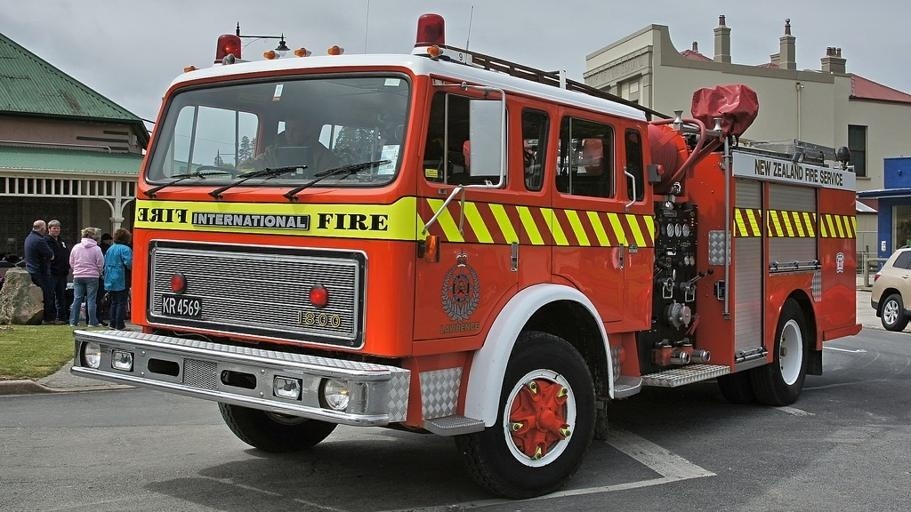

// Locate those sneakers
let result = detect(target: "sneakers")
[41,319,132,331]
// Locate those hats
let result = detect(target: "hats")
[101,233,112,240]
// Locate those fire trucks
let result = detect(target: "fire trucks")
[66,13,861,502]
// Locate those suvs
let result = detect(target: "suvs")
[870,245,911,332]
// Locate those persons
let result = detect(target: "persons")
[25,219,60,324]
[69,228,104,327]
[255,108,338,180]
[46,220,70,324]
[104,227,134,331]
[87,233,109,326]
